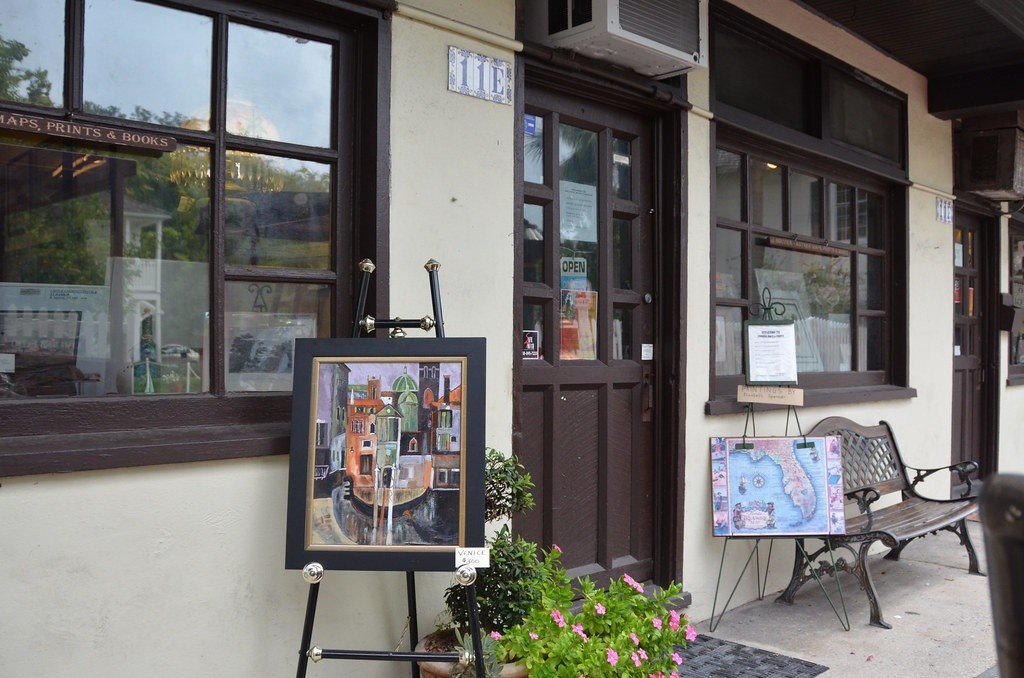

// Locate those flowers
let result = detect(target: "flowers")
[490,542,696,678]
[790,256,853,310]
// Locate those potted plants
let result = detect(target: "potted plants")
[414,445,539,678]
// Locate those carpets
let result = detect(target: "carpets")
[676,633,830,678]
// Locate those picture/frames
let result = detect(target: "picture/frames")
[285,337,486,572]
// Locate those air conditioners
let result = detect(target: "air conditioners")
[522,0,705,82]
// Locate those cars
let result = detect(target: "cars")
[161,345,200,365]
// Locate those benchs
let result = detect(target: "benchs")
[774,416,987,630]
